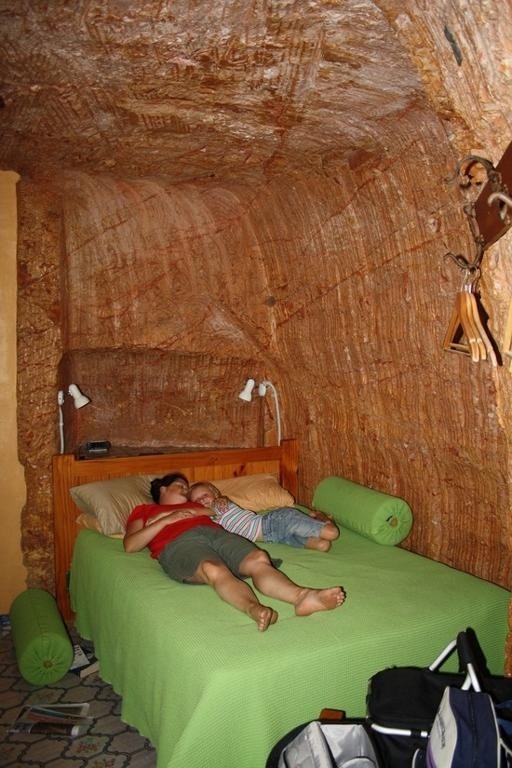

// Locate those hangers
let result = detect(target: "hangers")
[441,265,498,366]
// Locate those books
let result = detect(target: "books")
[68,646,101,679]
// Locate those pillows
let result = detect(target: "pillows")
[67,474,296,538]
[10,589,74,685]
[312,476,414,548]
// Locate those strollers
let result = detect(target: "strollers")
[266,626,512,768]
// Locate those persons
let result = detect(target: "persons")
[122,472,347,632]
[145,480,340,552]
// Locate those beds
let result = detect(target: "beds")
[51,439,512,768]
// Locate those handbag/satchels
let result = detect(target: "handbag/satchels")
[266,626,512,768]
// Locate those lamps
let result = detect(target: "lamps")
[57,384,91,454]
[237,379,282,446]
